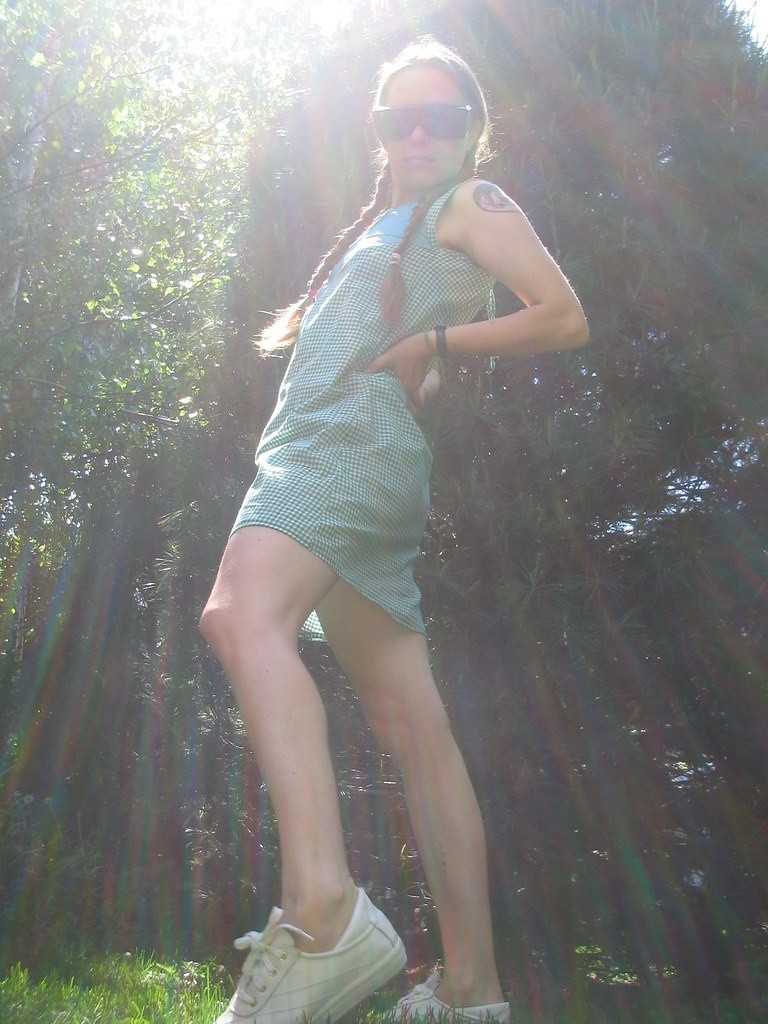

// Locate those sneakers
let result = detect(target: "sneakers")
[384,972,510,1024]
[212,889,409,1024]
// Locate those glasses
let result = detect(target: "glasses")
[368,102,475,143]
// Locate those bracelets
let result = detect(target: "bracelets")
[423,330,438,357]
[432,324,449,361]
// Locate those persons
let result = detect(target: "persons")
[196,42,589,1024]
